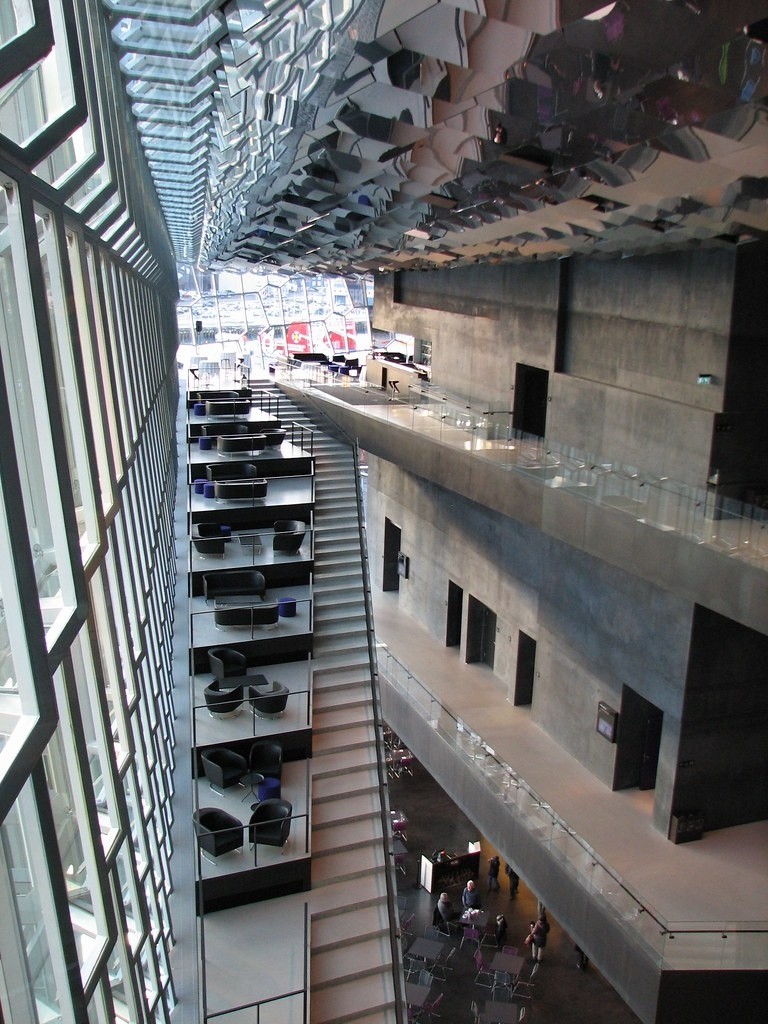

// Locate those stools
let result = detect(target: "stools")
[194,403,206,415]
[220,525,231,542]
[194,479,208,494]
[329,364,339,375]
[258,777,281,802]
[339,366,351,375]
[199,436,212,449]
[278,597,296,617]
[204,483,215,497]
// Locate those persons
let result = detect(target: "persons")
[462,880,480,913]
[530,912,550,963]
[486,855,519,900]
[574,944,589,970]
[437,892,462,923]
[432,848,447,863]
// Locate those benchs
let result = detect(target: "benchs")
[206,462,257,480]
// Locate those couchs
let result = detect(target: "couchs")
[192,523,225,560]
[214,597,279,631]
[201,424,248,444]
[217,434,266,457]
[260,428,287,449]
[197,390,239,403]
[373,352,405,364]
[208,647,246,678]
[201,748,248,797]
[203,570,266,605]
[291,353,329,366]
[249,680,289,719]
[205,399,250,420]
[249,740,282,783]
[204,680,244,720]
[214,478,267,503]
[249,798,293,855]
[273,520,306,554]
[192,807,244,865]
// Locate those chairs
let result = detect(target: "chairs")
[394,853,407,875]
[344,358,358,366]
[392,820,408,841]
[332,355,345,362]
[398,896,539,1024]
[383,726,414,779]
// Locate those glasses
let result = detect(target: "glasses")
[444,897,448,899]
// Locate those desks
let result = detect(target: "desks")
[390,809,409,826]
[405,981,431,1015]
[220,351,236,366]
[489,951,525,985]
[483,1000,517,1024]
[458,908,491,930]
[399,909,406,918]
[385,748,410,764]
[406,936,446,962]
[190,356,208,368]
[198,359,220,387]
[240,773,264,802]
[332,361,345,366]
[393,839,408,854]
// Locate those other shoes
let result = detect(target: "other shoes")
[532,959,543,962]
[510,896,515,901]
[577,963,587,968]
[516,891,518,894]
[487,887,499,892]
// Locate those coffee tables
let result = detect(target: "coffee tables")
[214,594,262,606]
[238,532,262,554]
[212,674,269,692]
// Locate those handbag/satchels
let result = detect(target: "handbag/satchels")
[576,945,582,953]
[525,935,533,944]
